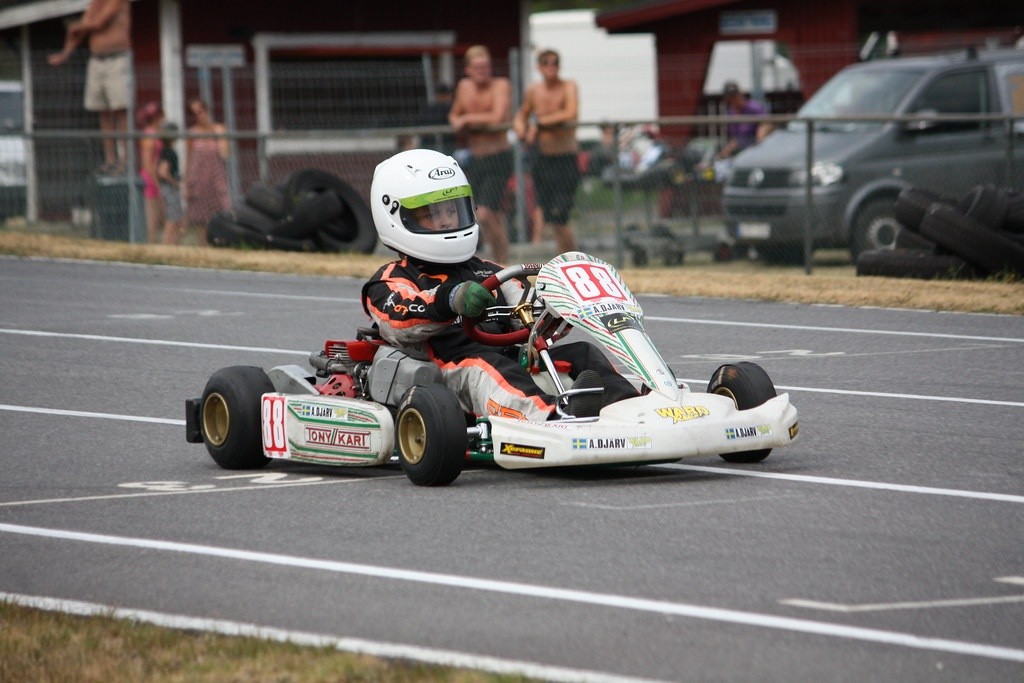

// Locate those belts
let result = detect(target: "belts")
[91,51,124,61]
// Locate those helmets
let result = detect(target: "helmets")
[370,149,478,264]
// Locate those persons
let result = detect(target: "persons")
[186,98,232,247]
[511,50,580,255]
[136,103,164,245]
[157,124,182,245]
[362,147,682,418]
[46,0,136,177]
[716,78,775,160]
[446,46,514,265]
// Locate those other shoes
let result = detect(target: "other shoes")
[91,163,127,178]
[559,370,601,419]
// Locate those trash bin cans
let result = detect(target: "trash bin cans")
[91,175,145,245]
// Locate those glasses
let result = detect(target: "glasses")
[542,58,559,67]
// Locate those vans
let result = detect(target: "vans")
[0,78,54,217]
[716,46,1024,264]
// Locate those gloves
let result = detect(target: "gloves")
[448,279,496,319]
